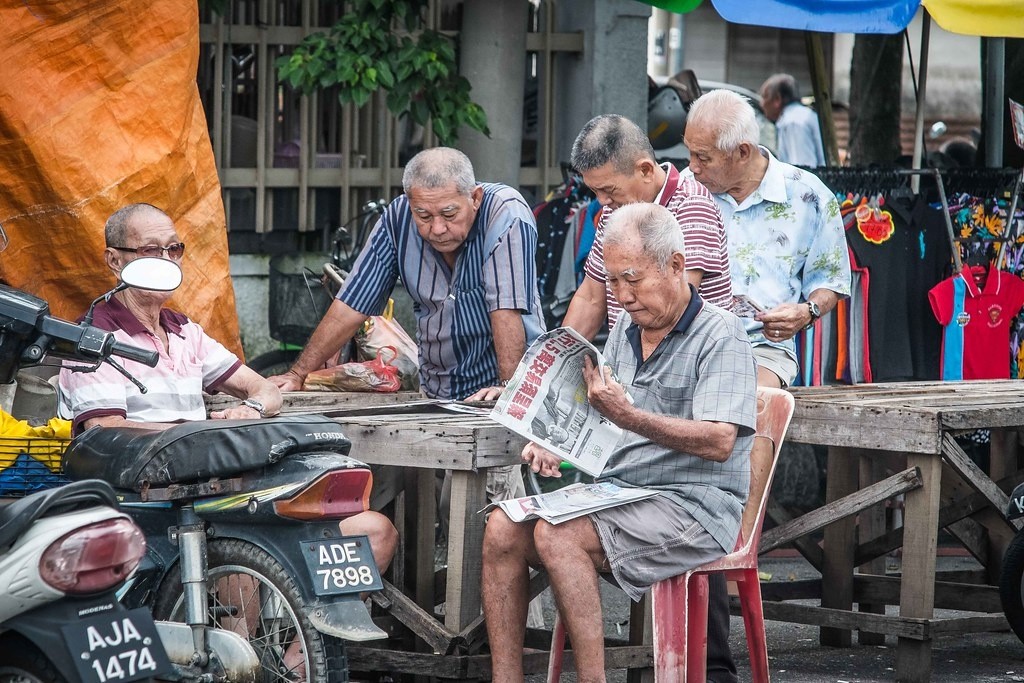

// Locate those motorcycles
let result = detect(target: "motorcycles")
[1,232,390,683]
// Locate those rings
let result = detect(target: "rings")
[775,330,780,337]
[496,388,500,391]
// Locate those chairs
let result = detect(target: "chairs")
[547,388,794,683]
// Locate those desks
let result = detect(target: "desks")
[204,396,656,683]
[721,380,1024,683]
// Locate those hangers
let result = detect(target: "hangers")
[964,237,989,287]
[795,163,1024,207]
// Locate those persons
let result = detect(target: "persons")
[56,203,399,683]
[265,147,549,653]
[680,88,851,547]
[572,113,737,683]
[756,72,826,169]
[532,397,568,444]
[480,202,755,683]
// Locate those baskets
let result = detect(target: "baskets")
[267,250,348,348]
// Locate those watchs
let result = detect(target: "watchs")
[242,399,264,417]
[806,301,820,325]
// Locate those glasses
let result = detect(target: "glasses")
[110,242,185,261]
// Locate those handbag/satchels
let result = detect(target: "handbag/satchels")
[302,343,401,393]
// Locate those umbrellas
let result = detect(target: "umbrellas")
[637,0,1024,193]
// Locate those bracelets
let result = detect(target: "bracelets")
[501,380,510,386]
